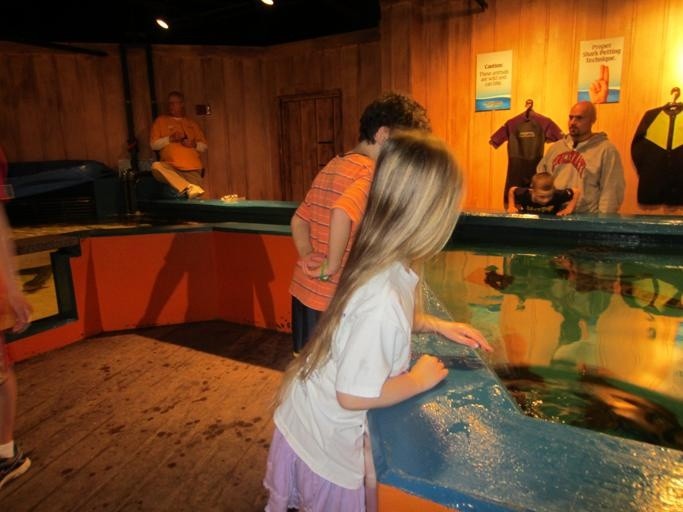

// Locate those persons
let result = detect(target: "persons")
[291,92,432,352]
[150,91,208,199]
[536,101,625,213]
[507,172,581,216]
[589,64,609,104]
[0,147,33,488]
[263,131,495,512]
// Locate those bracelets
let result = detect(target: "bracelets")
[320,260,328,281]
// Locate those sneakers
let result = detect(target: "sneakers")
[0,443,32,489]
